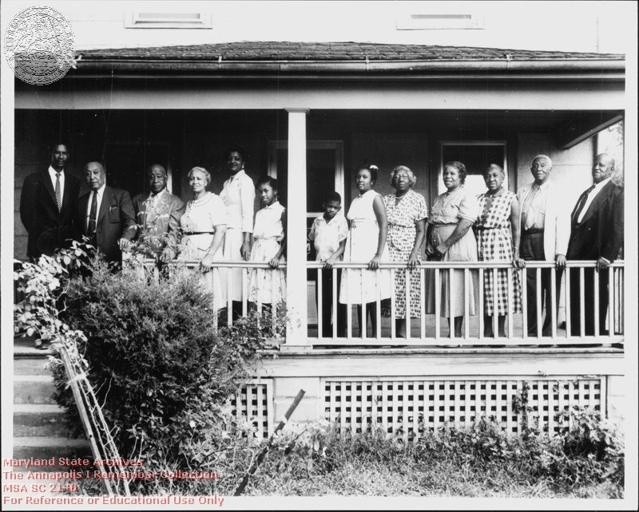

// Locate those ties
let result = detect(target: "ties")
[573,183,595,223]
[87,192,97,231]
[54,171,62,208]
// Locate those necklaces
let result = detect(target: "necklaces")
[395,195,405,199]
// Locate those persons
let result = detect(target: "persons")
[383,163,428,348]
[252,176,287,335]
[75,161,138,275]
[133,164,185,278]
[215,146,255,324]
[20,139,80,264]
[427,159,482,349]
[180,167,227,318]
[472,164,526,347]
[517,155,566,348]
[307,193,348,337]
[566,153,623,347]
[339,161,388,349]
